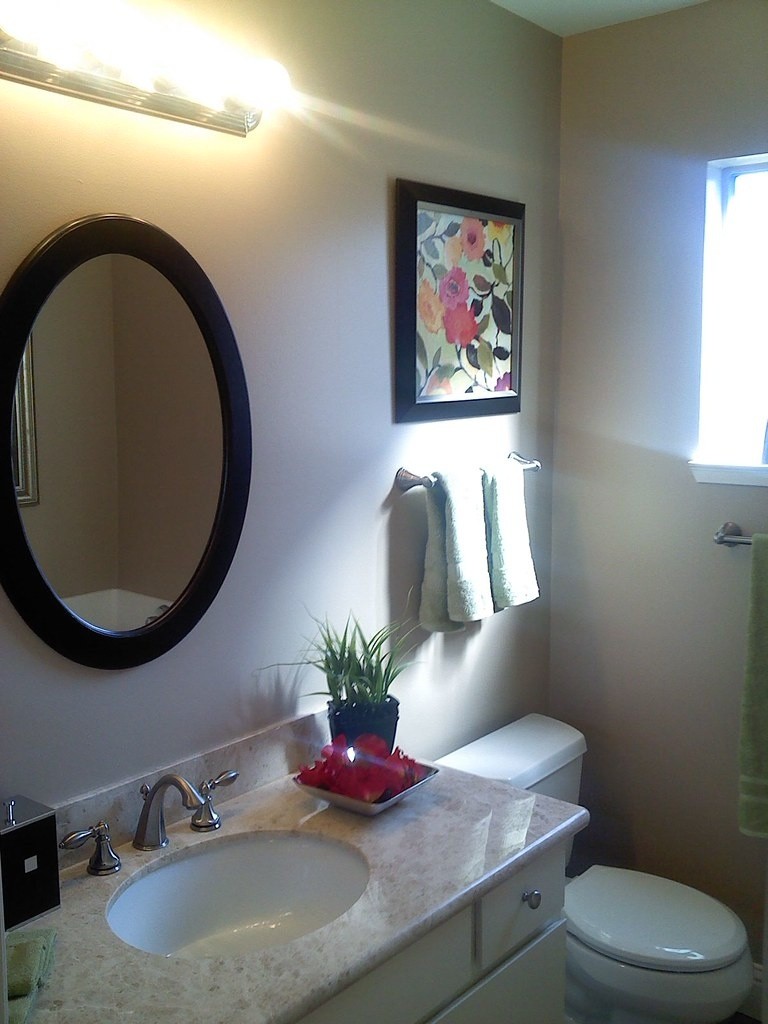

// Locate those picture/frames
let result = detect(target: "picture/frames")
[11,330,39,507]
[391,177,527,424]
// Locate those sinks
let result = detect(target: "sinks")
[102,827,373,961]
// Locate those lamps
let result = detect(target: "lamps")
[0,0,294,138]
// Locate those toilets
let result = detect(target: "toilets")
[430,710,757,1024]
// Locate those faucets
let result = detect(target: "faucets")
[131,772,206,851]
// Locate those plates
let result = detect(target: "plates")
[293,758,439,816]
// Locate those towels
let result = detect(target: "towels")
[735,532,768,839]
[417,457,543,633]
[4,927,59,1024]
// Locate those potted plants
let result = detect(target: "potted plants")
[291,610,425,760]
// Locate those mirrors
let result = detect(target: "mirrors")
[0,210,254,670]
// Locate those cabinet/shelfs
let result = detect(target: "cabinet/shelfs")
[292,833,568,1024]
